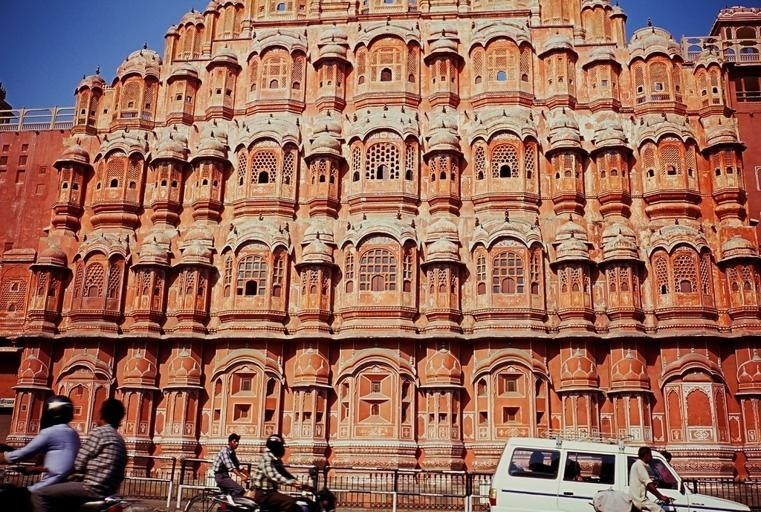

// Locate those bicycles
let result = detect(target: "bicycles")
[183,477,255,512]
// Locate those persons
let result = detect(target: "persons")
[31,398,127,512]
[0,395,80,493]
[248,434,302,512]
[629,447,668,512]
[214,433,249,498]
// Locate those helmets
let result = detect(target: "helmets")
[40,394,73,431]
[266,435,285,458]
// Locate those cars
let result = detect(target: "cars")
[487,436,751,511]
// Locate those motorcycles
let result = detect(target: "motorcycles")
[0,445,135,512]
[206,465,338,512]
